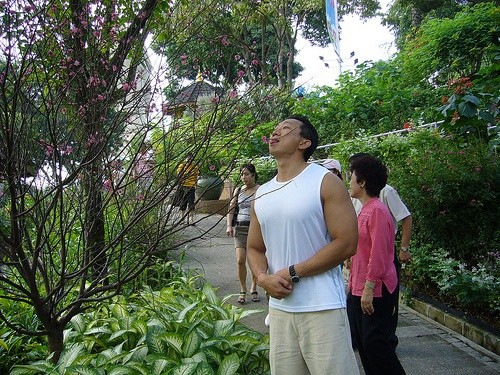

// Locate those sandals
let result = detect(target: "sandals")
[250,290,260,301]
[237,292,246,303]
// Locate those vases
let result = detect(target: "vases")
[196,175,224,200]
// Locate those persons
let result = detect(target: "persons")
[226,162,262,302]
[247,113,358,375]
[179,155,198,225]
[138,141,153,187]
[318,159,344,268]
[347,153,412,347]
[349,156,406,375]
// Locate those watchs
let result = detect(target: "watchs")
[290,265,300,283]
[401,246,408,252]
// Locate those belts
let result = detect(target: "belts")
[236,221,250,226]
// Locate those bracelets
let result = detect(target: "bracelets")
[365,284,374,289]
[363,291,374,295]
[227,225,232,227]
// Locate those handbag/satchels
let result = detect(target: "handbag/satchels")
[170,189,184,206]
[231,206,239,227]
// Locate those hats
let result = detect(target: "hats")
[318,159,342,174]
